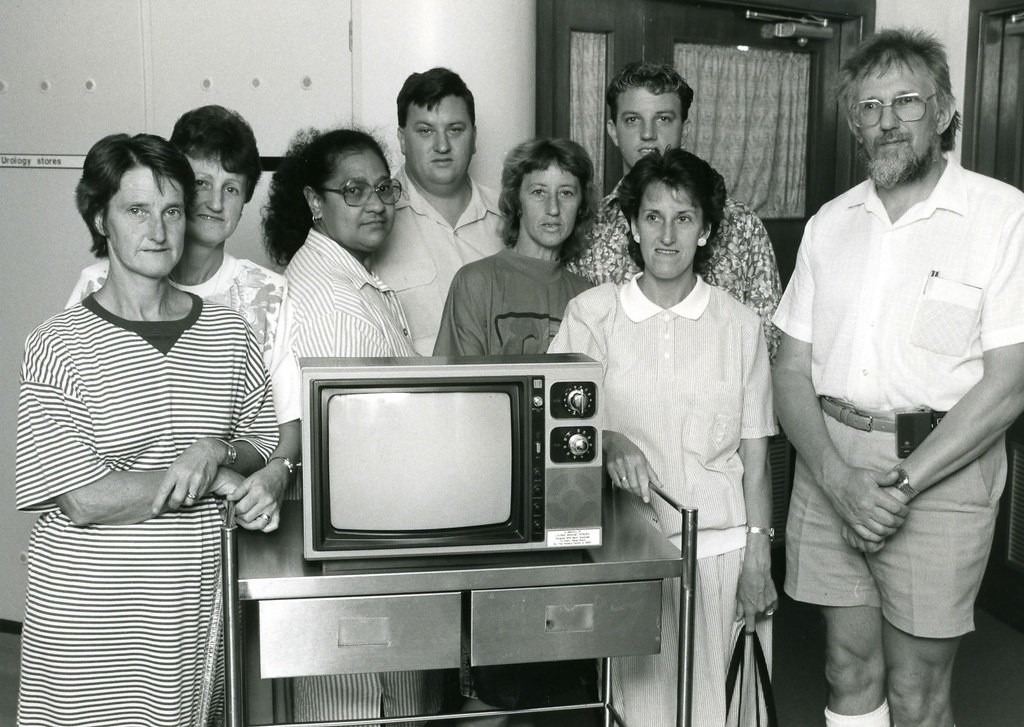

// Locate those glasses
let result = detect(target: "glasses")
[848,91,939,129]
[312,179,403,208]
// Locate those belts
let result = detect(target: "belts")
[820,396,895,434]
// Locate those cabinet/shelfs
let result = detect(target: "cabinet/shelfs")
[211,466,700,727]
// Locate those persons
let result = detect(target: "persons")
[366,63,529,356]
[259,126,426,359]
[741,28,1024,727]
[529,144,787,727]
[565,56,784,368]
[62,103,306,532]
[15,130,279,725]
[429,129,602,353]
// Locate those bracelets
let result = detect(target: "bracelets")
[264,453,296,478]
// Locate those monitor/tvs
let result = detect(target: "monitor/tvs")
[297,353,603,561]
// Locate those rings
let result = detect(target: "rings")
[619,476,626,483]
[187,491,198,499]
[258,511,273,524]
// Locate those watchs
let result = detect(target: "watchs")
[886,463,920,499]
[214,436,235,465]
[747,525,774,540]
